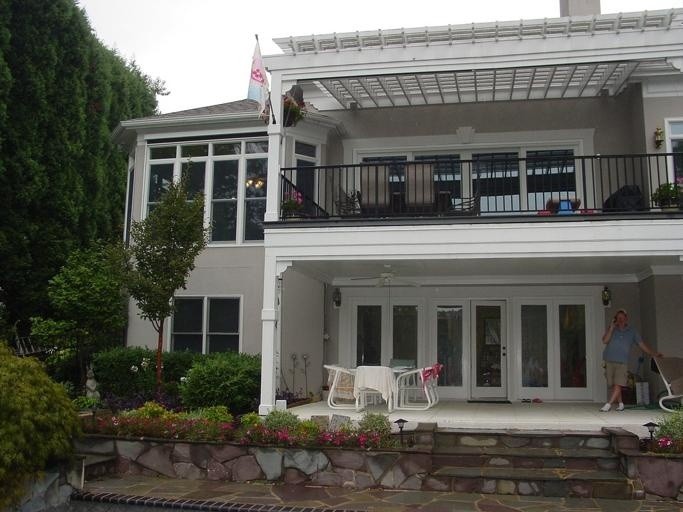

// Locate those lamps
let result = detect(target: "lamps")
[602,286,610,305]
[333,288,341,306]
[654,128,664,149]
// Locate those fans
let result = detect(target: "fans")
[351,273,418,288]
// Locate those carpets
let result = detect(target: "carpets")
[467,400,512,404]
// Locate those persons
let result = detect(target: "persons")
[598,309,664,412]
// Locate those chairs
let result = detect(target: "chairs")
[653,356,683,414]
[324,359,443,414]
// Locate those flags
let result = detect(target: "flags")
[248,38,270,114]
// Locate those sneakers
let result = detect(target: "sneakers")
[600,405,610,412]
[616,406,624,411]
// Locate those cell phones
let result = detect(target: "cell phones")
[613,317,617,323]
